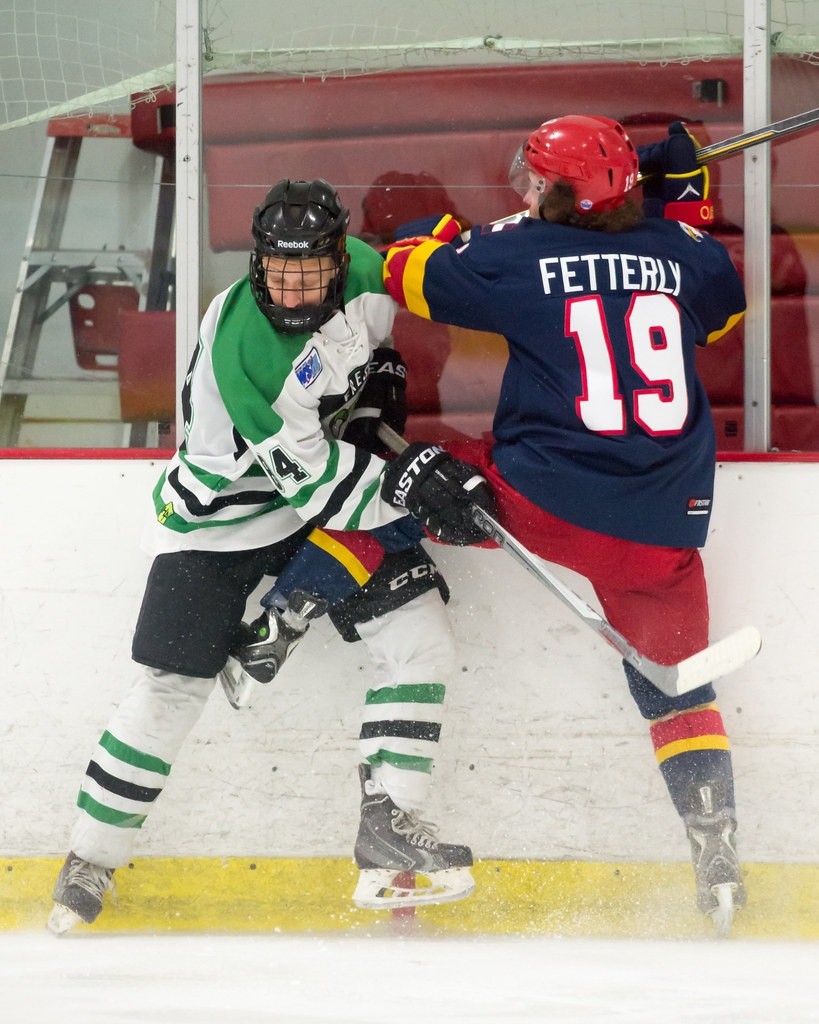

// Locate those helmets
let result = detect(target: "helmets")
[523,114,639,215]
[249,177,351,336]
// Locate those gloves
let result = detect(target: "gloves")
[380,442,497,546]
[380,214,461,244]
[636,121,715,227]
[344,347,408,453]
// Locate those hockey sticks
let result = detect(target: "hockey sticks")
[378,421,770,702]
[377,106,818,260]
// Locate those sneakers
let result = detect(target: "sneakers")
[217,590,328,710]
[47,851,117,934]
[352,762,476,909]
[684,776,748,935]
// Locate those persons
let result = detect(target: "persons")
[352,168,492,469]
[615,110,819,456]
[53,176,499,924]
[226,113,752,920]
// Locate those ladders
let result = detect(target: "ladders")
[0,111,173,447]
